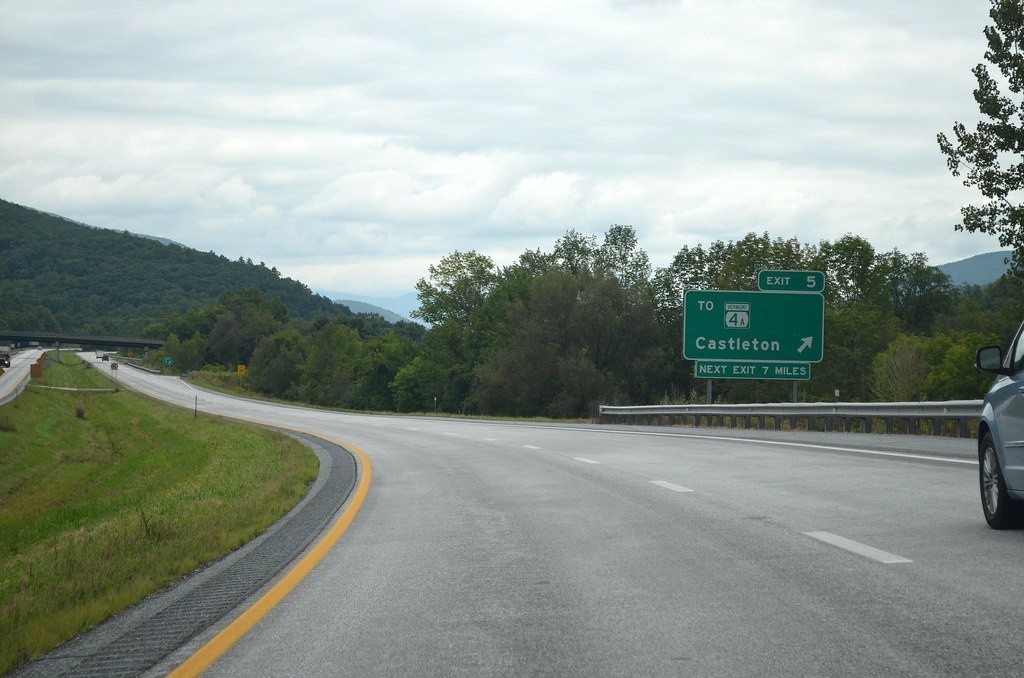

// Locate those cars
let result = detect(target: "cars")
[102,354,109,362]
[974,321,1024,530]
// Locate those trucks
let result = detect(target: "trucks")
[96,350,104,360]
[0,345,12,368]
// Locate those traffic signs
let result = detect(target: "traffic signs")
[682,288,824,363]
